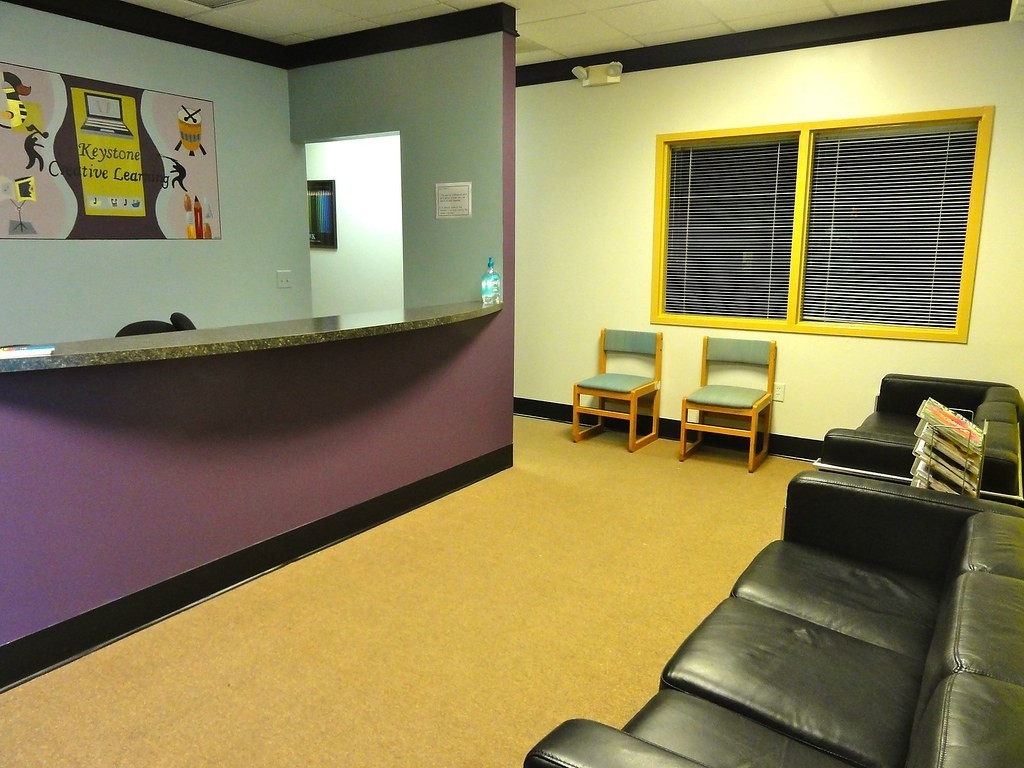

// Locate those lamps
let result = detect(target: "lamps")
[607,62,623,77]
[571,66,588,80]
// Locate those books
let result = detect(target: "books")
[915,397,983,495]
[0,346,56,356]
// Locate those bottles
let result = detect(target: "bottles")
[481,257,501,304]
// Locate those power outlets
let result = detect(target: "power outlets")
[772,382,785,403]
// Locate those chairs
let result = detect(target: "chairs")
[679,336,777,473]
[574,328,664,453]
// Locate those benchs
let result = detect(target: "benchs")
[821,373,1024,508]
[523,470,1024,768]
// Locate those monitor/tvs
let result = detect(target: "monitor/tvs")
[306,180,338,249]
